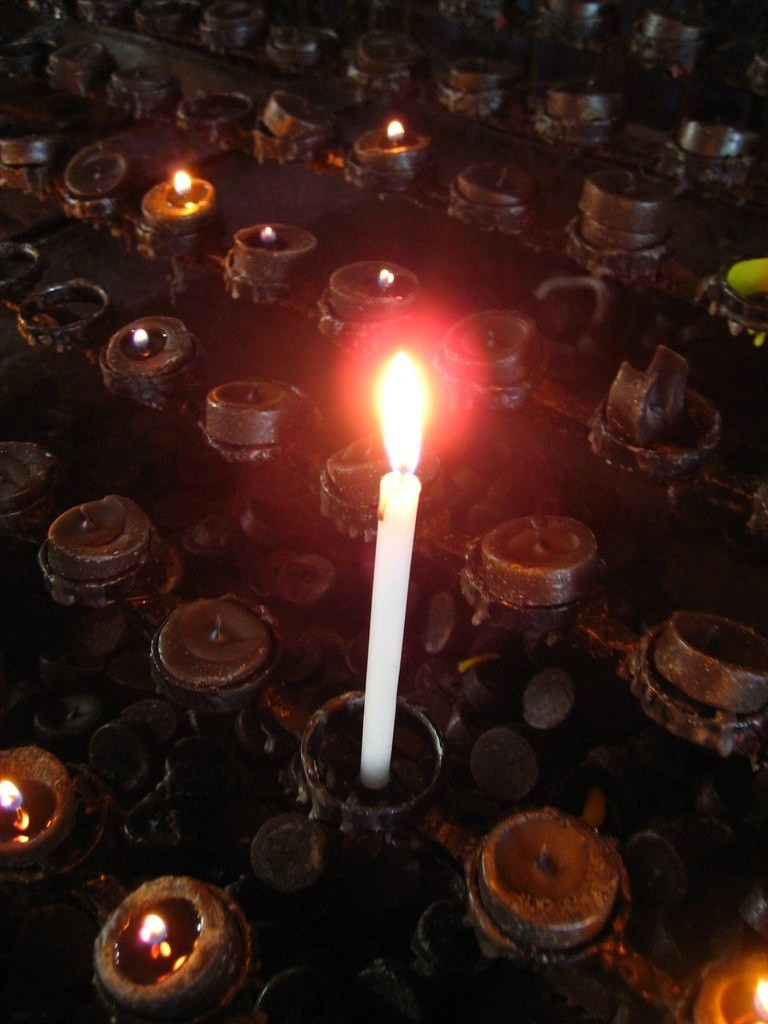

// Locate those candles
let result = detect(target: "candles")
[754,976,768,1022]
[360,339,437,792]
